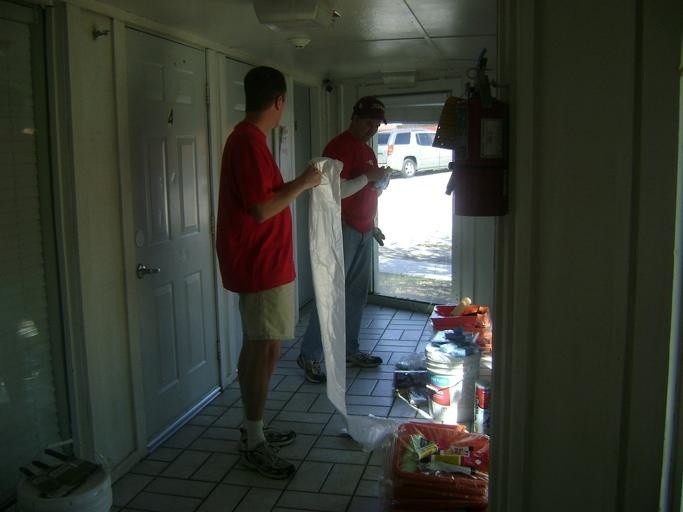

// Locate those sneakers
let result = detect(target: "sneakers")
[240,437,296,481]
[296,352,325,383]
[346,351,382,368]
[238,428,296,448]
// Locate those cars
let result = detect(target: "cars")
[377,129,452,178]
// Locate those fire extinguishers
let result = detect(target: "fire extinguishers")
[454,46,510,217]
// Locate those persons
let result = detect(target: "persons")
[215,66,322,479]
[295,96,392,385]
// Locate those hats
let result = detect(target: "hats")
[353,95,387,125]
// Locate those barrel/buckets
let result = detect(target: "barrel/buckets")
[17,437,113,512]
[470,356,493,437]
[423,342,481,424]
